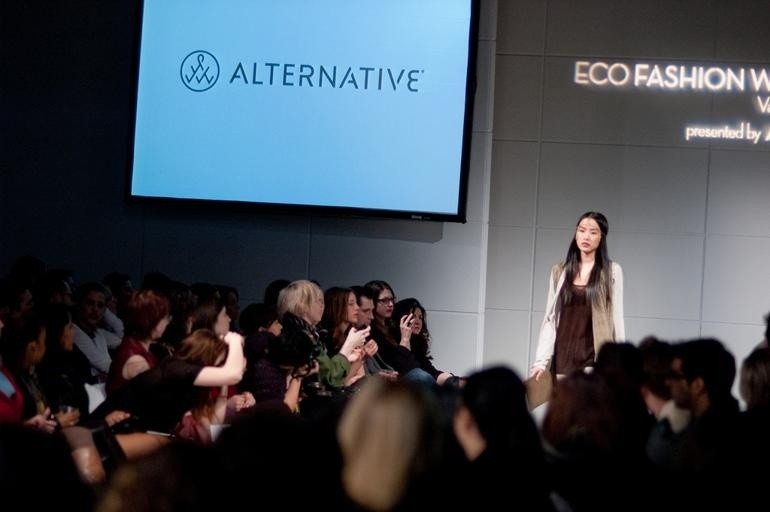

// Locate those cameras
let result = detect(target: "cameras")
[291,344,321,378]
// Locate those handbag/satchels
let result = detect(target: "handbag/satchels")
[525,368,555,411]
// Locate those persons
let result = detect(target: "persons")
[0,249,770,511]
[530,210,625,384]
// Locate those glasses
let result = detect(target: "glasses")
[377,298,395,305]
